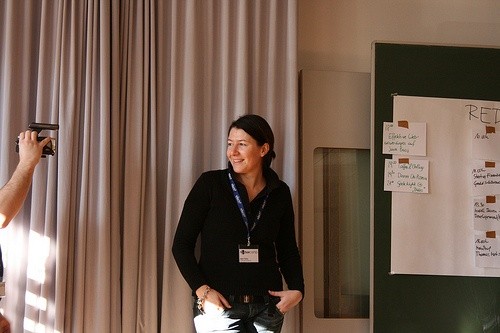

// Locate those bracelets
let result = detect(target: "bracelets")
[197,286,211,310]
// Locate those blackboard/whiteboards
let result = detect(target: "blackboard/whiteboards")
[370,41,500,333]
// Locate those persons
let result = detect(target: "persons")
[0,130,51,228]
[172,115,305,333]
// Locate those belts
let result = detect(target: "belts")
[195,295,280,304]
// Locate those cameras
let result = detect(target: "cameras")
[15,122,59,158]
